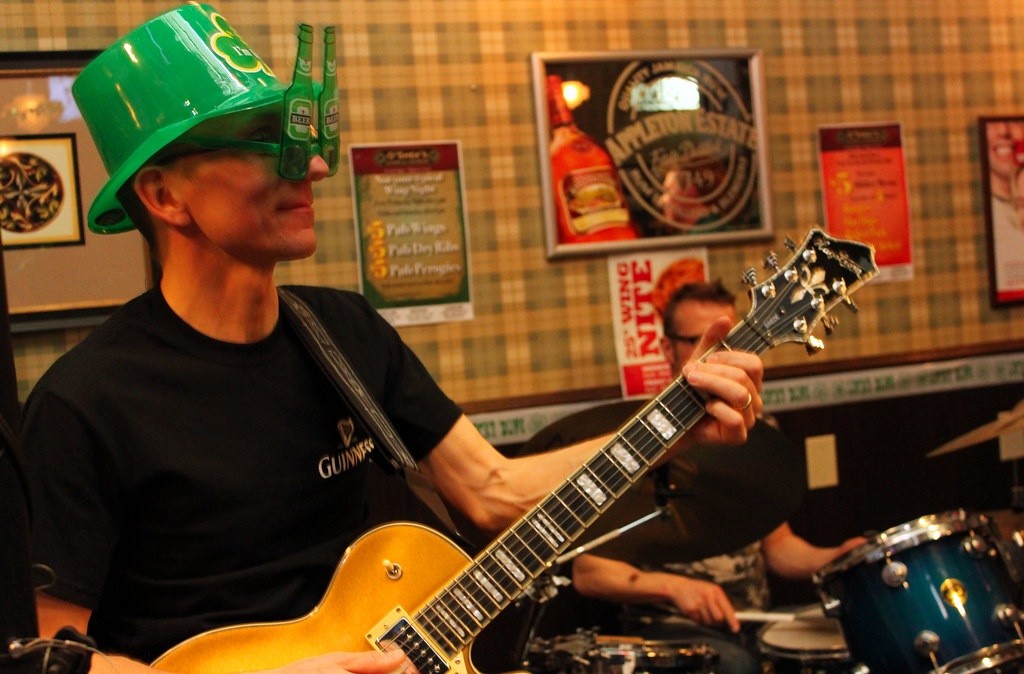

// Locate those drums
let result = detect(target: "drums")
[811,506,1024,673]
[523,623,721,674]
[755,599,858,674]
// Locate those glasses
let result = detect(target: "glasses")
[669,336,698,346]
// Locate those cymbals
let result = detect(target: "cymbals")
[517,397,798,563]
[922,402,1024,460]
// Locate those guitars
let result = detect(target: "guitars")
[141,223,885,673]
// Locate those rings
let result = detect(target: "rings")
[734,392,753,410]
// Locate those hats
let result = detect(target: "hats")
[71,2,327,235]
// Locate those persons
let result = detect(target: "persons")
[20,3,761,674]
[989,122,1024,290]
[571,281,867,660]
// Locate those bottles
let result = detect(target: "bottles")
[546,74,638,244]
[318,26,341,177]
[278,22,315,181]
